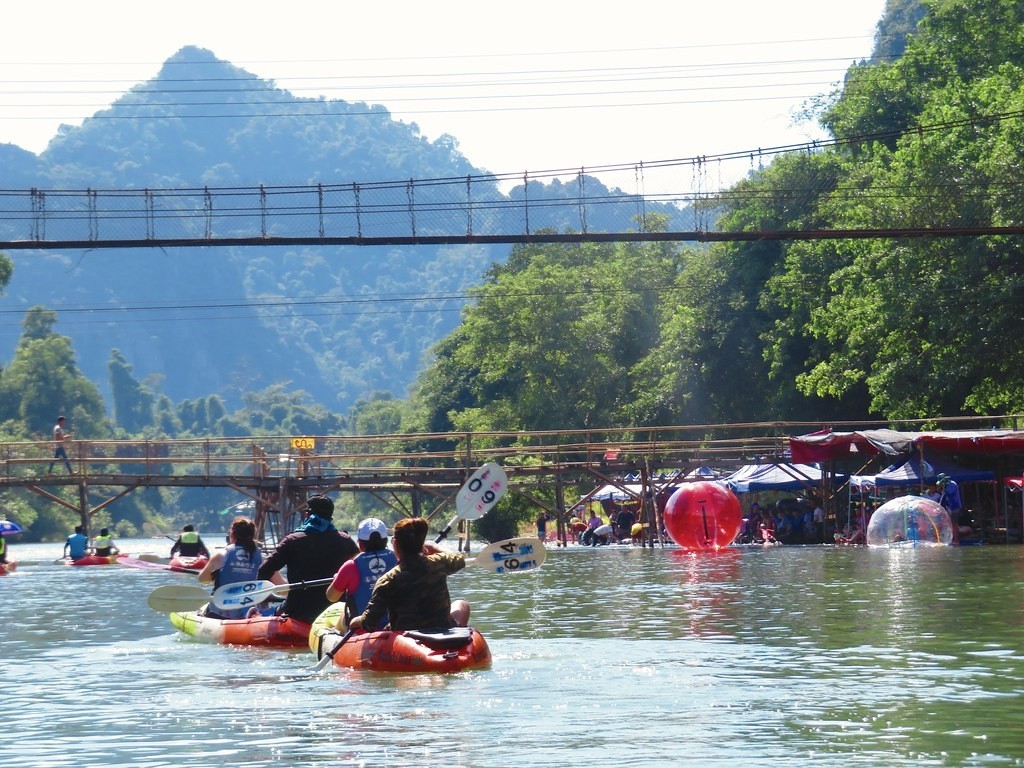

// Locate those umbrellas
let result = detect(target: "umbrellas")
[0,521,24,535]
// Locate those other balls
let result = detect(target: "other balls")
[865,494,954,548]
[663,480,743,550]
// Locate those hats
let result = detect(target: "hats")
[307,494,334,516]
[358,518,387,540]
[936,472,950,485]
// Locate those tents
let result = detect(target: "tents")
[788,426,1024,546]
[579,448,850,518]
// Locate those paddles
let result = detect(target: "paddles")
[212,537,548,611]
[309,460,510,674]
[39,555,70,566]
[116,556,202,576]
[146,584,287,613]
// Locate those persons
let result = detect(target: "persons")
[94,527,119,557]
[936,472,961,545]
[256,493,360,623]
[324,518,397,631]
[47,415,78,476]
[536,511,550,543]
[197,515,288,619]
[64,526,88,560]
[568,510,868,545]
[348,516,471,631]
[0,533,7,562]
[169,524,209,557]
[922,482,941,502]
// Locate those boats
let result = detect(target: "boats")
[68,553,119,565]
[0,560,16,575]
[170,601,312,647]
[169,552,209,569]
[307,600,492,674]
